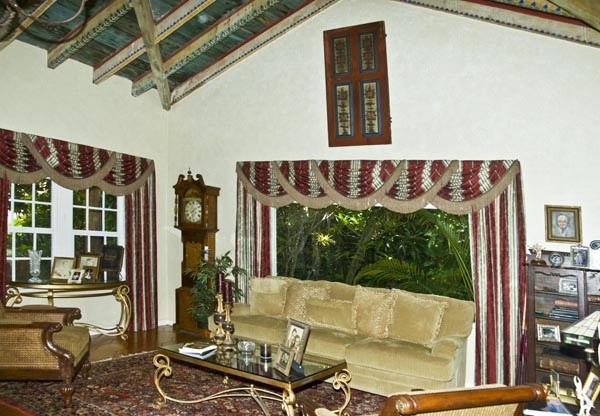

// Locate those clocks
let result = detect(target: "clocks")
[549,253,564,265]
[182,198,202,224]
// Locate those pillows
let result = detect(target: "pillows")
[248,277,285,316]
[305,297,356,333]
[351,283,396,338]
[388,288,447,346]
[280,279,330,322]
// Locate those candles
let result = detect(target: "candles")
[222,279,233,302]
[216,272,225,293]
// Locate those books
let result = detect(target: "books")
[178,339,218,359]
[548,298,580,322]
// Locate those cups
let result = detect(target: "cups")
[260,344,271,360]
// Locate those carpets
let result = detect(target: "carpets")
[0,351,386,416]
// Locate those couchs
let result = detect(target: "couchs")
[206,276,475,398]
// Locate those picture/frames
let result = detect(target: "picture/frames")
[537,324,561,343]
[571,245,589,267]
[282,318,310,364]
[575,366,600,408]
[75,252,101,279]
[82,265,96,284]
[559,278,577,294]
[50,257,76,285]
[273,344,294,376]
[68,269,84,284]
[546,205,581,243]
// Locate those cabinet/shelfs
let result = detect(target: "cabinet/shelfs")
[525,261,600,388]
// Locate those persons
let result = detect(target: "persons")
[291,335,301,351]
[290,328,297,343]
[86,258,95,266]
[56,260,71,278]
[556,213,574,236]
[281,354,285,363]
[84,268,93,280]
[284,353,289,364]
[73,272,79,280]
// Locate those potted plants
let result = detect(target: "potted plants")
[182,250,248,339]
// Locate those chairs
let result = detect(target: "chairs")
[376,373,544,416]
[0,300,91,408]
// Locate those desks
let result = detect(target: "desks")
[5,281,132,340]
